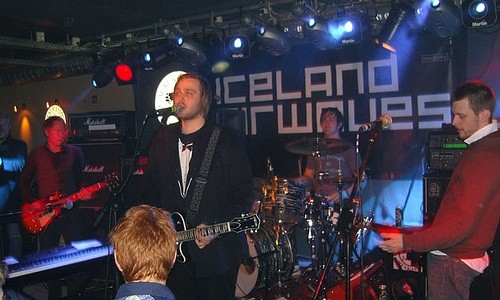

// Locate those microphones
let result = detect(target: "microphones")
[148,105,181,117]
[47,201,73,209]
[358,114,392,133]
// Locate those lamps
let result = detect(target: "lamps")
[91,0,500,87]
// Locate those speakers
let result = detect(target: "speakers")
[71,141,142,285]
[422,171,500,300]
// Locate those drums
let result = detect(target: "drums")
[235,215,294,300]
[303,196,340,230]
[260,181,306,224]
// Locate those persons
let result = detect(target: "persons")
[0,112,95,300]
[378,80,500,300]
[105,204,181,300]
[136,74,256,300]
[288,107,367,261]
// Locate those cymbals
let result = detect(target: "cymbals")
[285,139,353,156]
[317,178,357,185]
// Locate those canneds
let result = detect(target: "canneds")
[396,207,403,228]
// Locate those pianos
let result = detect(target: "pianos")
[0,238,114,300]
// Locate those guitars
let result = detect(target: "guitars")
[171,212,261,264]
[355,215,433,275]
[20,172,120,236]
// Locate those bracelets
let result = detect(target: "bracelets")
[343,188,349,198]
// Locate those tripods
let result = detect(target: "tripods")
[288,154,348,300]
